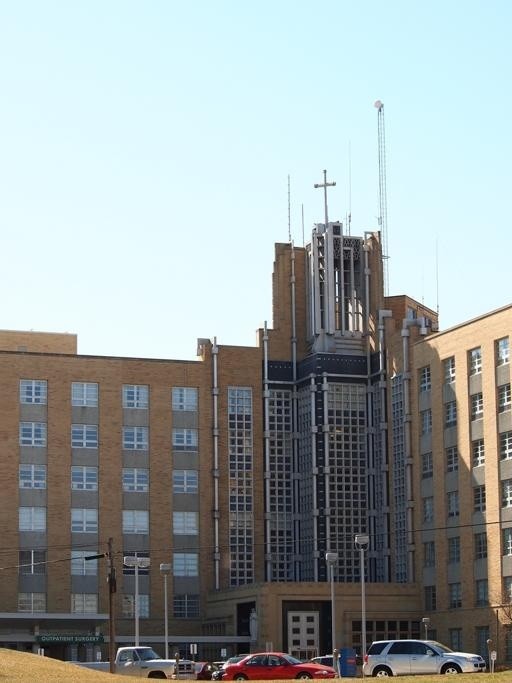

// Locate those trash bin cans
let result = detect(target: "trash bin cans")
[339,648,356,677]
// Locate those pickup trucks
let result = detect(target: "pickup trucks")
[75,646,196,680]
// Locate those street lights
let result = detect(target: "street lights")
[421,618,434,638]
[326,552,339,678]
[160,562,174,660]
[354,534,371,674]
[124,552,151,646]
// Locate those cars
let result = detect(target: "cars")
[193,660,220,681]
[221,653,341,681]
[363,638,486,677]
